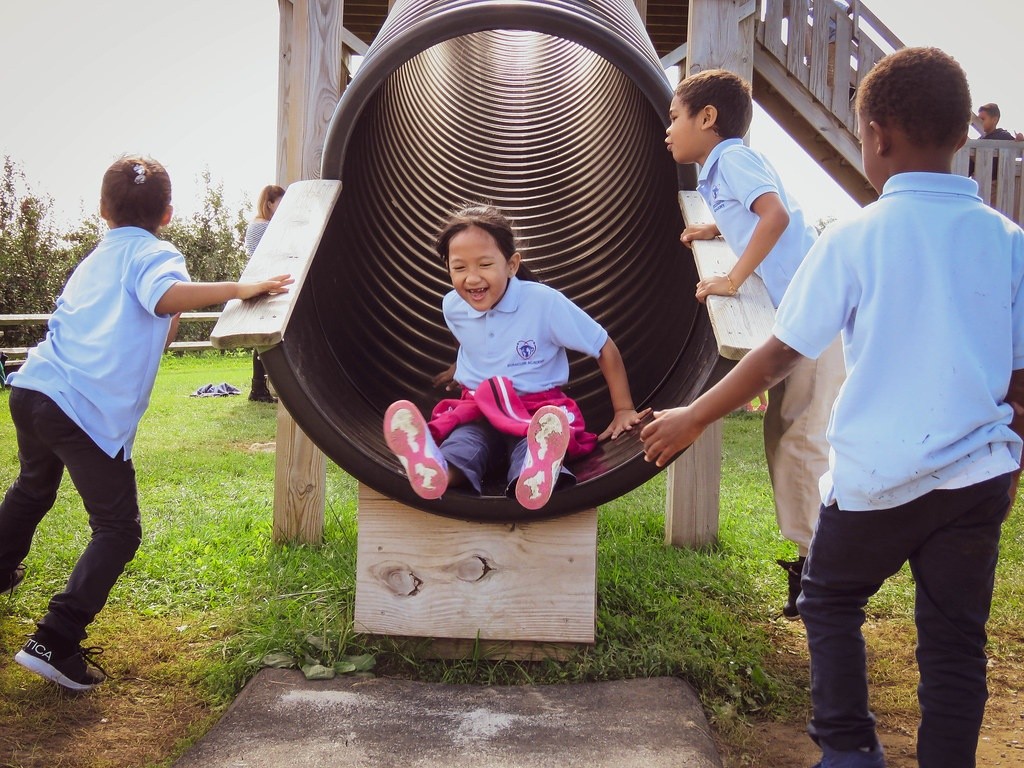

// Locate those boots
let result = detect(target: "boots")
[250,379,278,403]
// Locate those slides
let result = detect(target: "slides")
[211,0,816,523]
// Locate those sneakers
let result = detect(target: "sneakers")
[517,406,570,509]
[15,630,136,689]
[813,736,884,768]
[385,400,447,499]
[777,560,801,620]
[0,564,27,600]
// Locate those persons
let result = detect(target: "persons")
[638,45,1024,768]
[663,68,848,619]
[807,0,859,104]
[379,205,653,510]
[246,184,288,403]
[967,102,1024,210]
[0,152,297,690]
